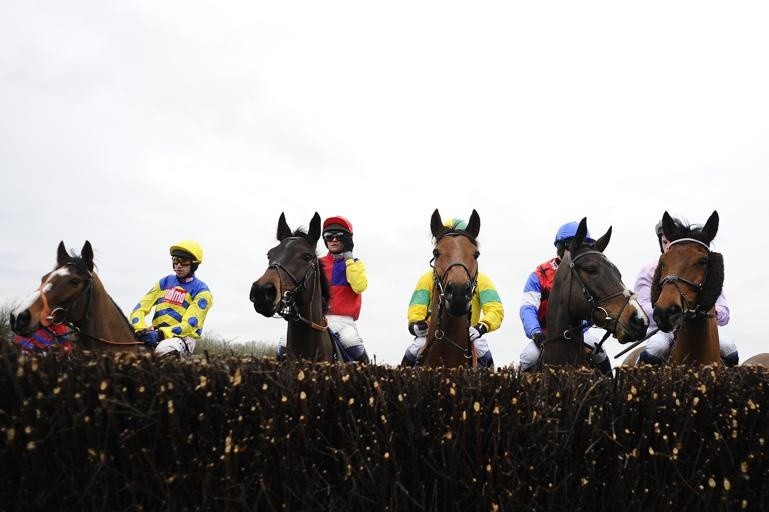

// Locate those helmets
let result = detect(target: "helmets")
[445,218,465,230]
[656,219,682,235]
[322,216,353,236]
[170,240,203,264]
[555,222,596,244]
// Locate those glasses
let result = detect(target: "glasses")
[324,232,346,241]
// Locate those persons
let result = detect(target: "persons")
[277,217,369,365]
[618,220,738,367]
[129,241,213,356]
[397,218,503,372]
[503,223,614,377]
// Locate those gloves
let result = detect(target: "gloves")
[344,238,354,260]
[469,324,486,341]
[534,333,545,348]
[136,329,164,346]
[410,321,430,338]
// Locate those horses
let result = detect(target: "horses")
[651,210,724,367]
[413,208,481,371]
[522,219,648,374]
[9,240,151,355]
[249,212,357,364]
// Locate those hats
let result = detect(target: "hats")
[172,257,201,265]
